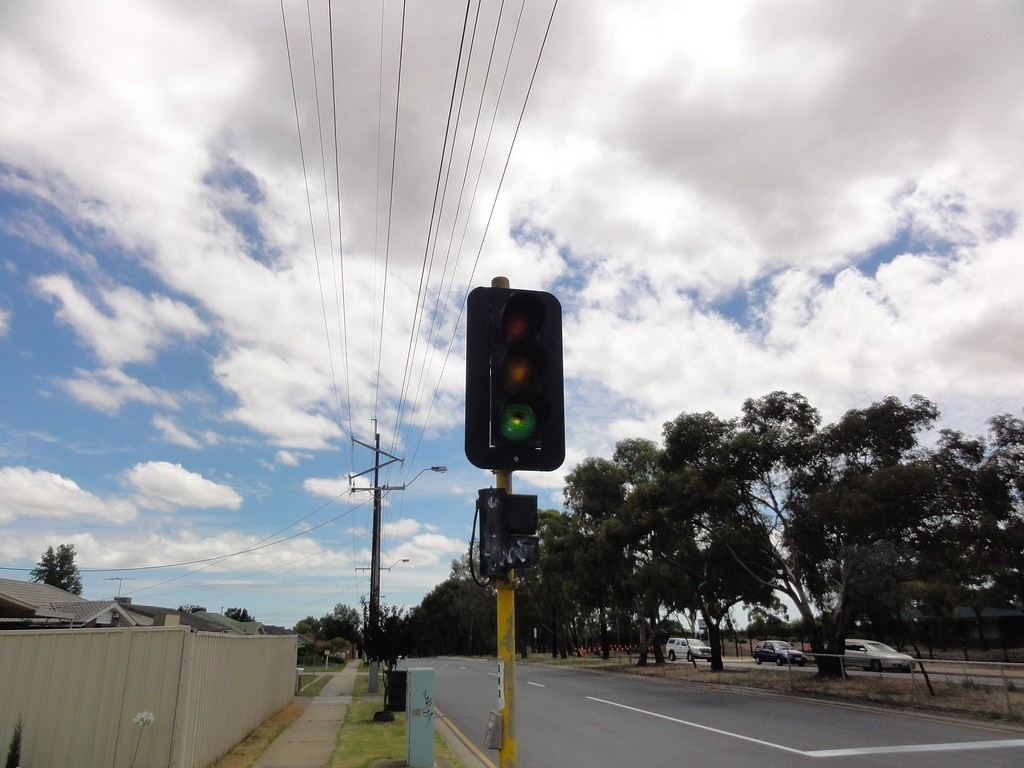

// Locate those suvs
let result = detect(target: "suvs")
[666,638,712,662]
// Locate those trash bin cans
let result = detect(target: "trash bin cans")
[382,667,408,712]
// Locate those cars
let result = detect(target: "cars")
[753,641,808,667]
[824,639,916,673]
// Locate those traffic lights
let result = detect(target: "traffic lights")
[464,287,565,472]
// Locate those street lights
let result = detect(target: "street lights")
[351,466,447,692]
